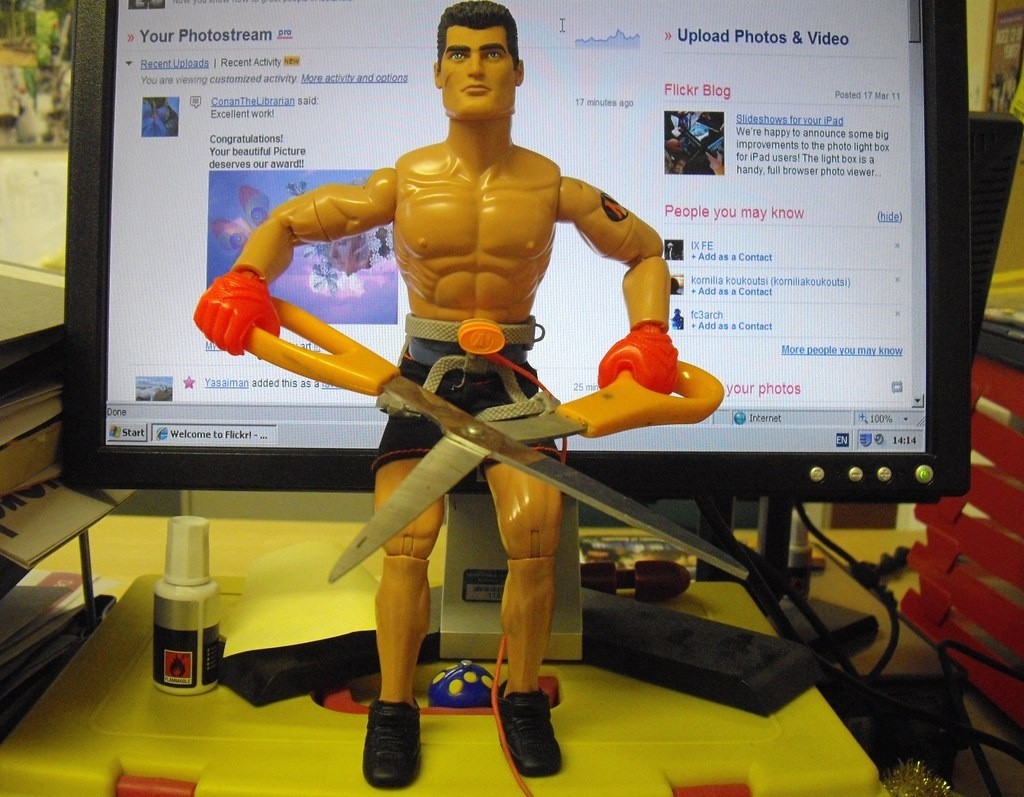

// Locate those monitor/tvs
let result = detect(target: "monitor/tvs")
[63,0,1023,719]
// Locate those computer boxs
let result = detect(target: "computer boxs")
[795,544,969,789]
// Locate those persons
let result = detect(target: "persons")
[194,2,679,789]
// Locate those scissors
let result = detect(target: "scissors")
[243,295,747,587]
